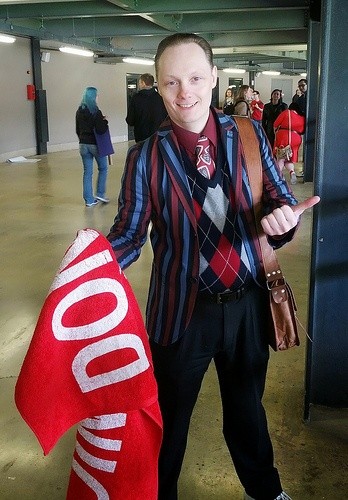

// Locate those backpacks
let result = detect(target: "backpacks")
[223,101,249,116]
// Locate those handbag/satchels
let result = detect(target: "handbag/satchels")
[275,145,292,159]
[92,109,114,156]
[268,279,302,351]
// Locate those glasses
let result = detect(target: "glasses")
[298,85,306,87]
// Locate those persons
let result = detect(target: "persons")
[75,87,110,207]
[262,89,288,161]
[222,88,236,114]
[249,91,265,125]
[288,78,307,177]
[104,32,320,500]
[125,73,167,144]
[232,85,253,116]
[273,103,304,185]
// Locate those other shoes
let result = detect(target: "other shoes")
[290,171,297,184]
[96,195,110,202]
[243,490,292,500]
[87,200,98,206]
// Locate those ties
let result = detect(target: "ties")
[196,137,216,179]
